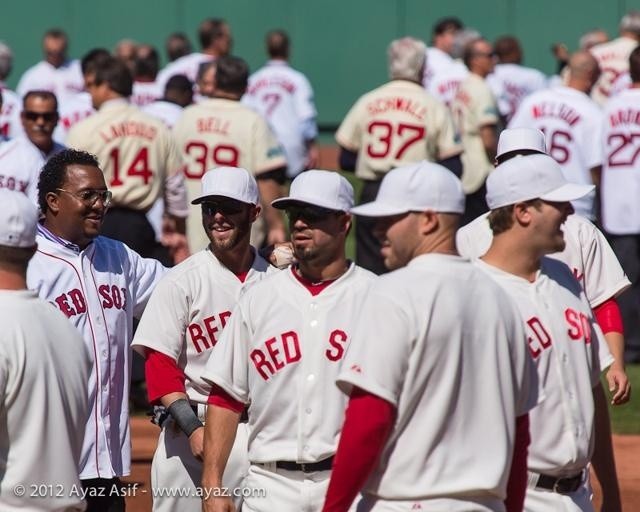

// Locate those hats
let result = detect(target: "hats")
[0,188,39,248]
[191,128,596,216]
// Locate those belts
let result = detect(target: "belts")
[536,471,583,493]
[260,456,332,472]
[187,404,249,423]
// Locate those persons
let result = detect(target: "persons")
[0,11,640,512]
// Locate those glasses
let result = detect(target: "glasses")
[201,198,244,215]
[55,188,112,208]
[25,111,55,121]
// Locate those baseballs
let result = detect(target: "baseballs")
[273,246,293,269]
